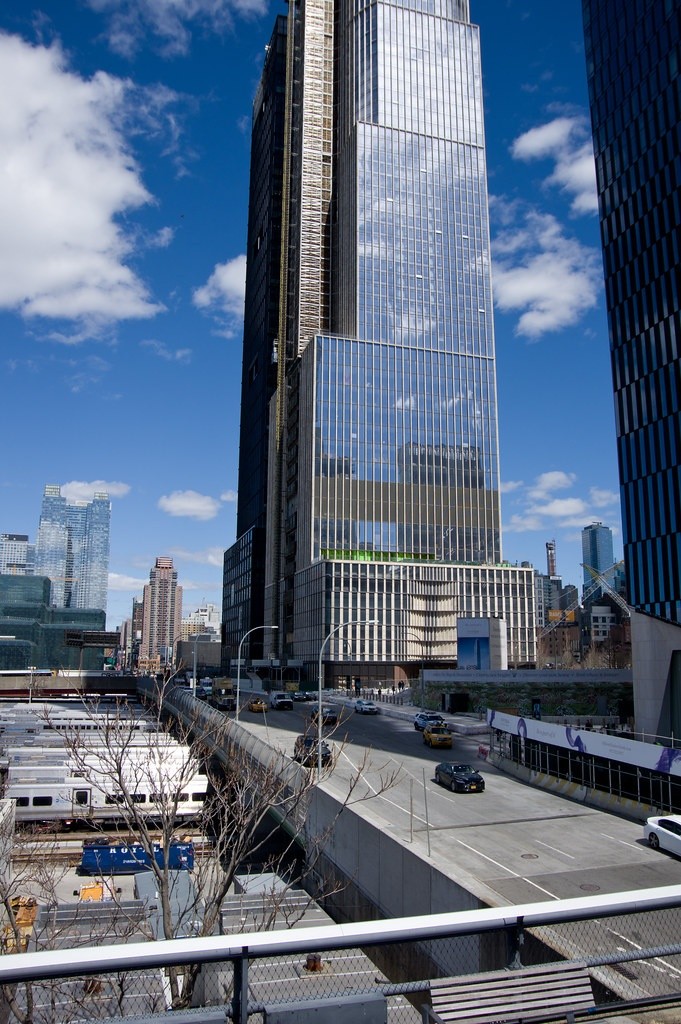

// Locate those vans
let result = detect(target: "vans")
[201,679,212,695]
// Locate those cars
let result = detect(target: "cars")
[196,687,206,699]
[248,697,268,712]
[232,685,241,695]
[304,692,316,701]
[173,673,199,684]
[435,762,485,792]
[292,692,306,701]
[643,814,681,856]
[311,706,337,724]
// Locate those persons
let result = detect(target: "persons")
[377,681,404,697]
[564,719,634,735]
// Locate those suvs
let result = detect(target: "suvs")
[423,724,452,749]
[271,693,293,710]
[355,699,378,715]
[294,735,332,766]
[414,711,447,730]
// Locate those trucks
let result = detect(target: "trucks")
[208,678,236,711]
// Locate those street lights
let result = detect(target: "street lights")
[193,627,213,697]
[398,632,426,712]
[235,626,279,722]
[172,633,193,683]
[320,619,378,786]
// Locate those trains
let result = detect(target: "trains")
[0,697,229,829]
